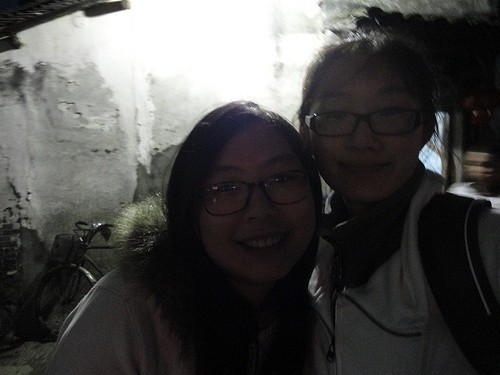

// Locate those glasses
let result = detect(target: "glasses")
[303,106,432,137]
[192,168,310,217]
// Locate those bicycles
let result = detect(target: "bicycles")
[33,220,117,341]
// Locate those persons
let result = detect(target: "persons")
[43,100,338,374]
[446,145,500,213]
[297,30,500,375]
